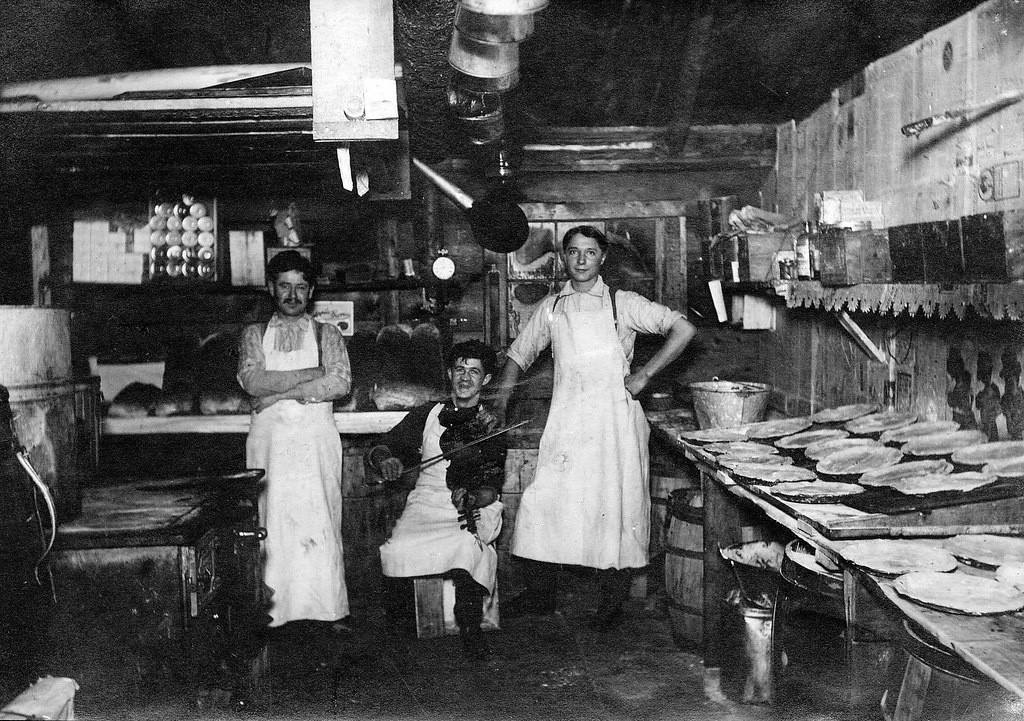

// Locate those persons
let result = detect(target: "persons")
[236,249,353,627]
[365,339,508,660]
[476,225,697,634]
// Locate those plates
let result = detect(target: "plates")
[433,257,455,280]
[679,403,1024,614]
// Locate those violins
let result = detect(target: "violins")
[438,421,488,535]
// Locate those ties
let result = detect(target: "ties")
[282,326,294,353]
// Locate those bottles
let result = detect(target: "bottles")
[779,220,890,285]
[149,193,214,278]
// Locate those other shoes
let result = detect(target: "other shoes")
[461,625,490,658]
[500,597,555,616]
[394,614,412,630]
[593,605,621,629]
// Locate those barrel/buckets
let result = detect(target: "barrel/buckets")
[667,487,706,649]
[648,462,696,553]
[716,598,773,703]
[767,539,895,721]
[690,377,772,431]
[883,616,1024,721]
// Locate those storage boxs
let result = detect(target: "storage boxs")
[820,209,1024,286]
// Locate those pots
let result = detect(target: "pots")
[715,539,785,608]
